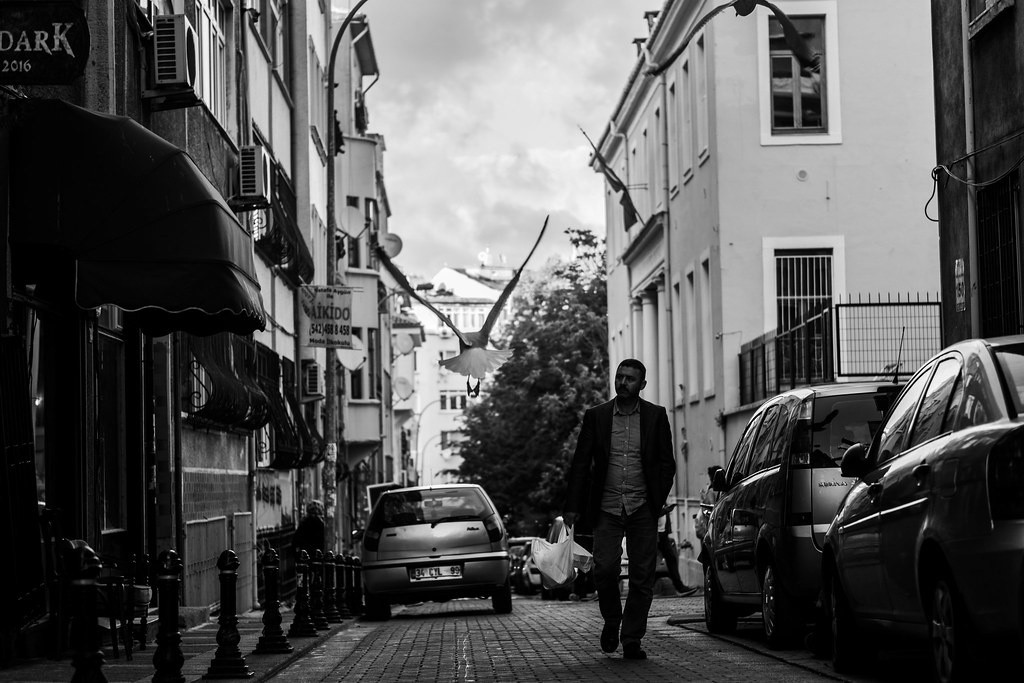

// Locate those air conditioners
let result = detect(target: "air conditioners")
[239,146,273,204]
[152,13,200,101]
[305,363,325,395]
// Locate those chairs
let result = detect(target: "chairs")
[56,538,154,660]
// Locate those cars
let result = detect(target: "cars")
[702,380,910,651]
[350,483,513,621]
[506,515,629,600]
[820,335,1024,683]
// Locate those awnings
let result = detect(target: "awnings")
[1,98,265,343]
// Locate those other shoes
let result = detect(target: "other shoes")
[679,586,697,597]
[623,641,647,659]
[600,614,621,653]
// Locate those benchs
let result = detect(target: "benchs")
[390,507,476,524]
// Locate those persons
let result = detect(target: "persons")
[657,502,698,596]
[293,502,326,557]
[563,359,676,659]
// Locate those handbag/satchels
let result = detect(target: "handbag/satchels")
[530,520,594,589]
